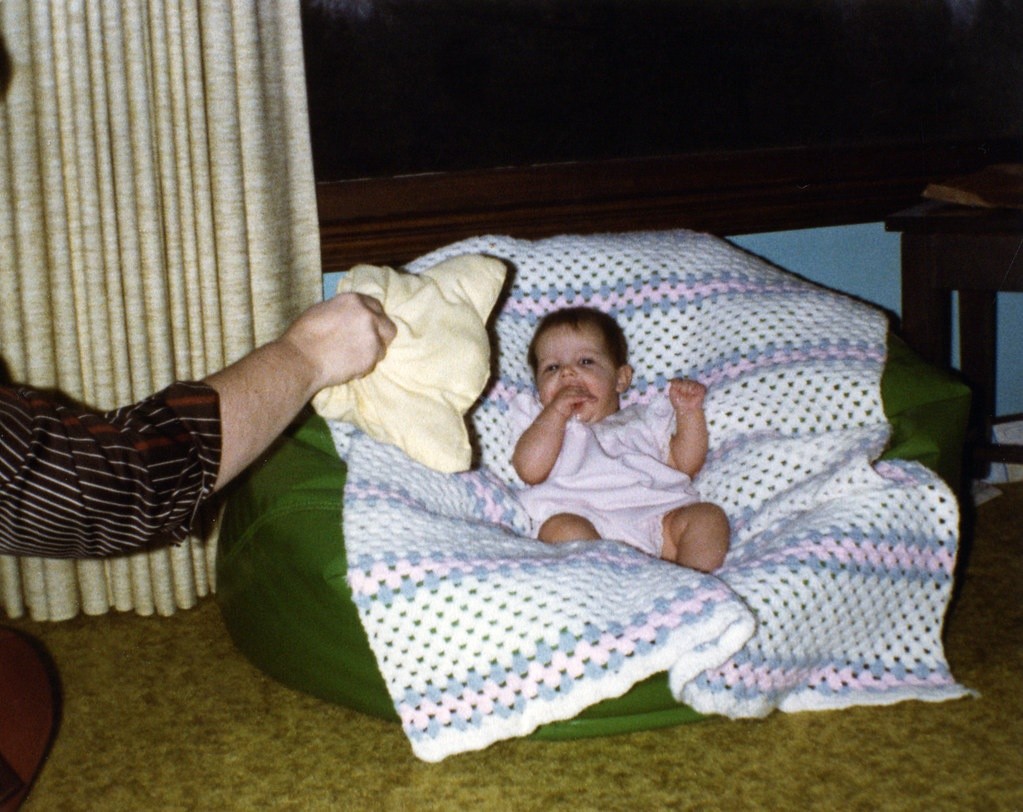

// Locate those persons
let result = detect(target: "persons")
[511,306,732,574]
[0,290,398,559]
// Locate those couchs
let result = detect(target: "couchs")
[218,223,985,762]
